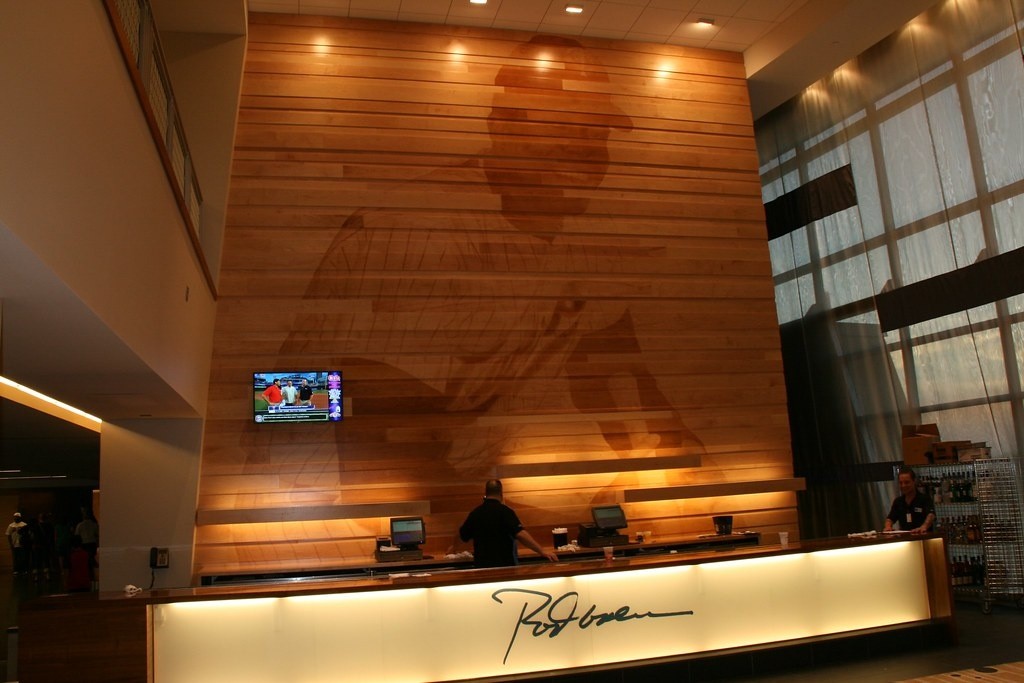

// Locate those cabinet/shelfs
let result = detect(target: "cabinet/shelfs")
[934,501,986,593]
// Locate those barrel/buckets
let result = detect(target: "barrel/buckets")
[712,516,732,535]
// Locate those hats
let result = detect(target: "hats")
[13,512,21,518]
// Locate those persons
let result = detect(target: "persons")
[297,379,314,406]
[281,380,299,406]
[459,479,559,568]
[882,469,937,535]
[5,504,99,593]
[262,379,286,406]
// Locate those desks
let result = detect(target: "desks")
[10,520,958,683]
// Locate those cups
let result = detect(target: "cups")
[603,547,613,563]
[552,532,568,549]
[636,531,651,543]
[778,532,788,545]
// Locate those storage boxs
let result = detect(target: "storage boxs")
[956,446,994,462]
[930,439,972,466]
[900,420,941,465]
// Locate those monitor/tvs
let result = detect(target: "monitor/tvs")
[390,517,425,544]
[592,506,628,530]
[252,371,344,424]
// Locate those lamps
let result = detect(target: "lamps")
[697,18,717,28]
[565,3,584,14]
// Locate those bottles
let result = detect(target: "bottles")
[917,471,978,504]
[937,515,983,544]
[949,554,985,587]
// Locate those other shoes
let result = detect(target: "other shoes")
[13,569,48,575]
